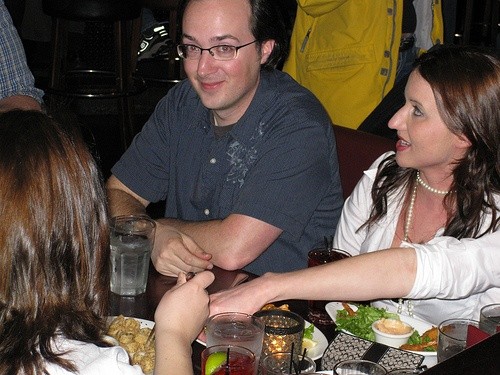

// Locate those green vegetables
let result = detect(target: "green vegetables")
[304,323,314,340]
[335,304,435,352]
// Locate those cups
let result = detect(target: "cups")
[201,344,256,375]
[437,318,496,363]
[307,248,353,325]
[479,303,500,335]
[261,352,316,375]
[333,359,387,375]
[109,215,157,296]
[205,312,265,375]
[371,320,414,348]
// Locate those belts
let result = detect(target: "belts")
[399,37,415,53]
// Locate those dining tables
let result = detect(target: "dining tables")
[108,253,342,375]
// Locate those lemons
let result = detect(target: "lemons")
[205,352,228,375]
[303,338,318,347]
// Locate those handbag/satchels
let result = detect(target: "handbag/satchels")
[321,330,427,375]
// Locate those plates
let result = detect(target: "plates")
[324,302,437,356]
[195,319,329,360]
[102,316,155,334]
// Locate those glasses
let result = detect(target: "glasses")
[177,36,265,61]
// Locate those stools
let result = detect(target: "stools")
[34,0,148,160]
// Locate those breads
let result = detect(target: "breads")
[262,304,289,359]
[107,314,155,375]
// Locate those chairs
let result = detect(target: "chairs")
[331,124,398,203]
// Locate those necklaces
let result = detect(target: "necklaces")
[403,174,418,244]
[417,171,460,195]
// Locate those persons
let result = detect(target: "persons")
[0,1,47,114]
[104,0,344,278]
[0,106,215,375]
[281,0,444,132]
[208,33,500,319]
